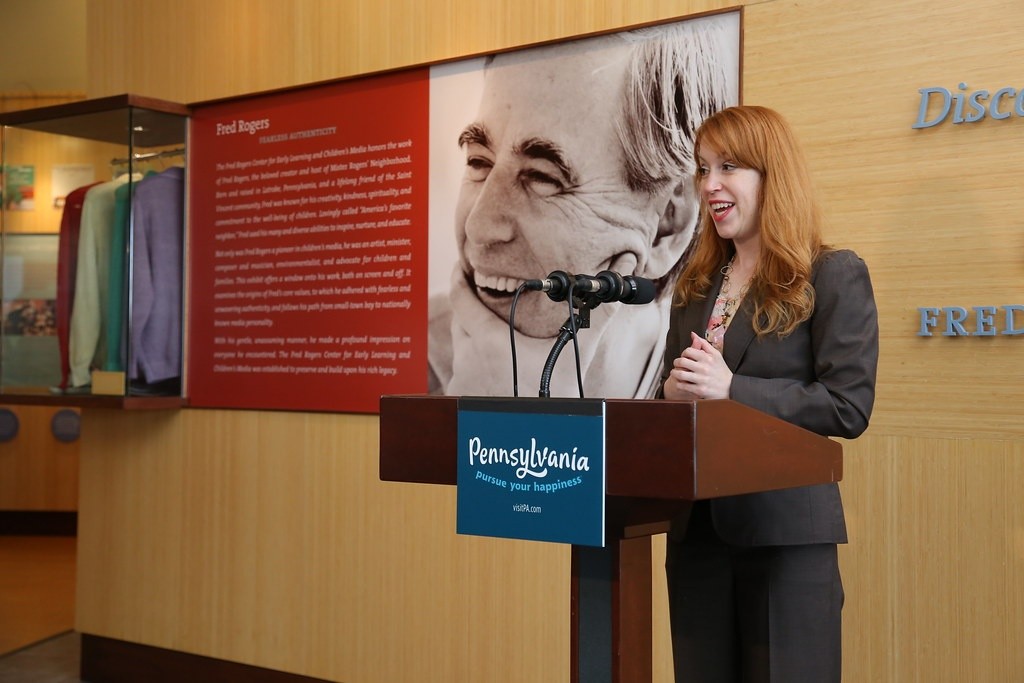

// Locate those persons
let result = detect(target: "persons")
[428,12,741,401]
[655,106,878,683]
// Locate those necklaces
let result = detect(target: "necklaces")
[722,254,758,299]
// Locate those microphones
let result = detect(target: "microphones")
[524,269,656,304]
